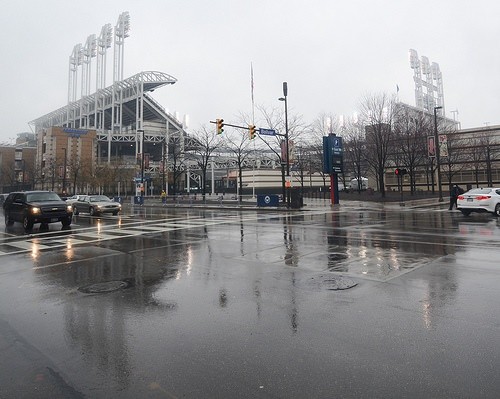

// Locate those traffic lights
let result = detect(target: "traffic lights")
[248,124,256,139]
[216,118,224,134]
[395,168,407,176]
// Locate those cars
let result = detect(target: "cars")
[62,195,122,216]
[457,187,500,216]
[0,193,9,206]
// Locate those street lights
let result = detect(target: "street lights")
[433,106,444,202]
[137,129,145,197]
[278,98,290,175]
[61,147,67,193]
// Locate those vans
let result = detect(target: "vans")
[345,177,369,191]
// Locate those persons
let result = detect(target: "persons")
[160,190,167,207]
[447,184,464,210]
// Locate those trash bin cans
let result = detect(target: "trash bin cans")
[134,197,142,204]
[113,196,122,203]
[291,193,303,208]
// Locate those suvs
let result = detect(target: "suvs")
[3,189,73,231]
